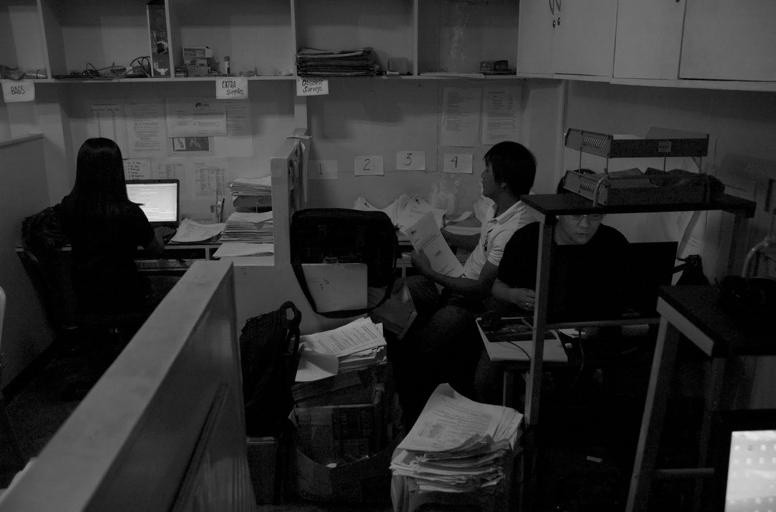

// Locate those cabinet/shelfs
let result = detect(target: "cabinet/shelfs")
[1,0,776,94]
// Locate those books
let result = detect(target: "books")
[171,176,272,260]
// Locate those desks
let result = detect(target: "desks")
[462,287,661,464]
[63,219,224,283]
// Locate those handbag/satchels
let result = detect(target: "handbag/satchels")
[289,207,398,288]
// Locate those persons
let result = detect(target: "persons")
[384,141,540,372]
[42,137,166,316]
[490,169,629,324]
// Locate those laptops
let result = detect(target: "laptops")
[123,179,180,246]
[523,240,678,322]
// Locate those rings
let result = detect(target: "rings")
[526,303,528,306]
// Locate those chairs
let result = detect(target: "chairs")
[19,244,138,358]
[294,206,398,329]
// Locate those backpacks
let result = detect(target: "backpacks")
[238,301,303,439]
